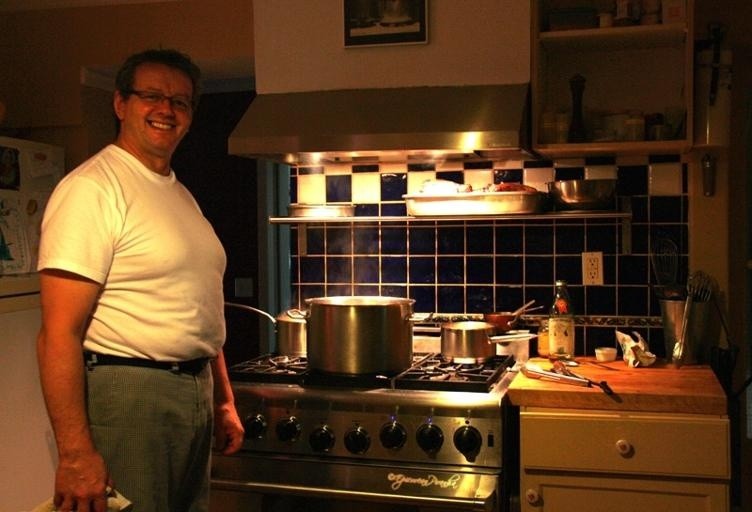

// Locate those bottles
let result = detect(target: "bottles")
[536,320,549,357]
[566,72,587,142]
[610,0,661,27]
[548,280,576,361]
[623,111,673,142]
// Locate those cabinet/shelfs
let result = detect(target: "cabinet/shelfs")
[516,402,734,511]
[522,0,699,164]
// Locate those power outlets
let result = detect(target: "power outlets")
[581,251,604,286]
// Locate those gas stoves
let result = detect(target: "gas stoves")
[224,349,514,468]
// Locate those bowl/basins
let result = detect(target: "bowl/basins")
[543,178,619,209]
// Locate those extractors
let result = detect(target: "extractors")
[227,1,534,158]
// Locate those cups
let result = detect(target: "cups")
[539,106,569,144]
[658,298,715,364]
[595,347,618,362]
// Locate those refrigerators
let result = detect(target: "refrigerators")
[1,135,66,511]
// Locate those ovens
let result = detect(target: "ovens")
[209,453,502,512]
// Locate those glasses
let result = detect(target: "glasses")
[128,89,190,112]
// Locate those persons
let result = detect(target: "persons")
[36,48,245,512]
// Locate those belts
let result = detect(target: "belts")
[86,350,210,372]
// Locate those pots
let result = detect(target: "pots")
[484,305,546,332]
[221,298,308,360]
[439,319,538,365]
[286,296,434,376]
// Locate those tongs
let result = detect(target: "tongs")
[520,361,591,389]
[671,293,693,370]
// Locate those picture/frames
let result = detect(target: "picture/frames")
[340,1,429,50]
